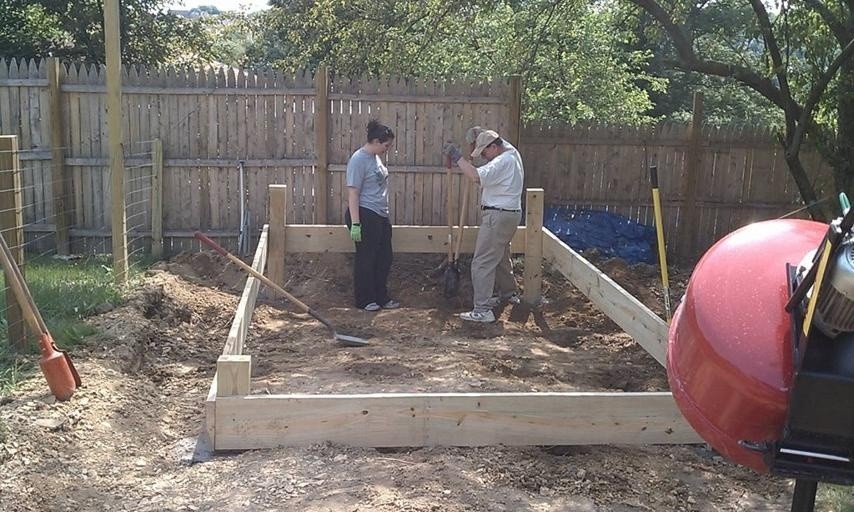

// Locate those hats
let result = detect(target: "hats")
[470,129,500,158]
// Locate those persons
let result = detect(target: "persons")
[343,119,399,311]
[442,126,524,323]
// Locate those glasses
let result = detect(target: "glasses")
[380,126,392,141]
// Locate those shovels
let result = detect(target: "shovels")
[192,231,370,346]
[443,140,475,298]
[0,233,82,401]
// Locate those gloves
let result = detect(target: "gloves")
[441,143,462,164]
[464,125,484,145]
[350,221,362,242]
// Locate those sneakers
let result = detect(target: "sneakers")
[364,302,381,311]
[381,298,400,309]
[492,292,521,305]
[459,309,496,323]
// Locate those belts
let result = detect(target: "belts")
[480,204,521,213]
